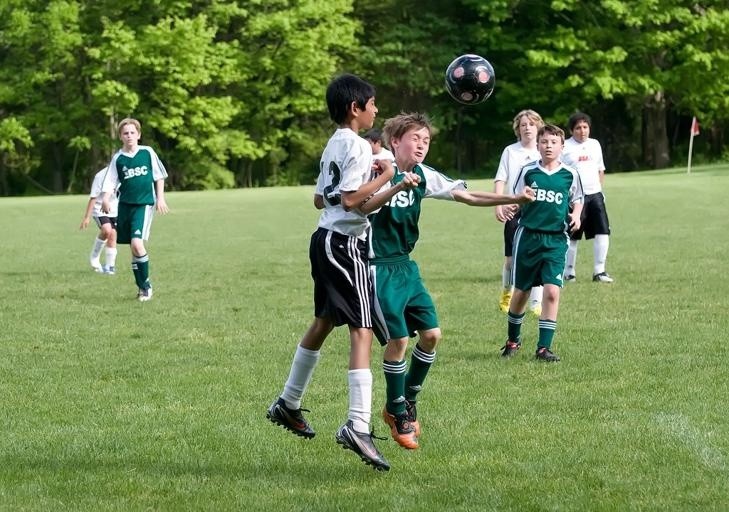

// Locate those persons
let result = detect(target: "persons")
[557,113,612,282]
[80,166,120,275]
[99,118,169,301]
[360,130,395,165]
[265,75,393,473]
[359,111,533,449]
[494,109,545,317]
[499,124,583,362]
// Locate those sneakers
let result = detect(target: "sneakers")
[137,284,152,302]
[266,396,316,440]
[90,256,103,273]
[563,266,577,283]
[384,400,420,449]
[536,346,560,362]
[335,419,390,472]
[528,300,542,317]
[500,285,511,314]
[103,265,115,275]
[500,337,522,358]
[593,272,613,283]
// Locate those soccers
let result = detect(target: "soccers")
[446,54,495,106]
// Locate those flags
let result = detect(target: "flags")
[687,116,699,174]
